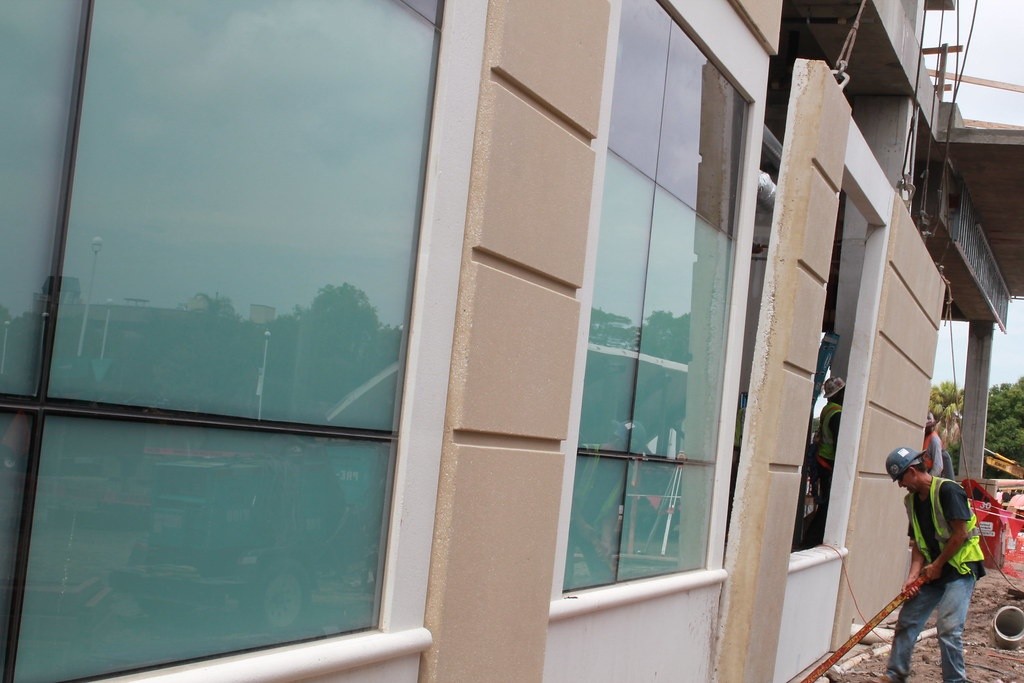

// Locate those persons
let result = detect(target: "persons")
[560,421,647,592]
[799,377,846,547]
[922,412,945,478]
[866,447,987,683]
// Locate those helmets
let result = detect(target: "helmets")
[823,377,846,400]
[613,418,649,453]
[926,411,937,428]
[884,446,929,483]
